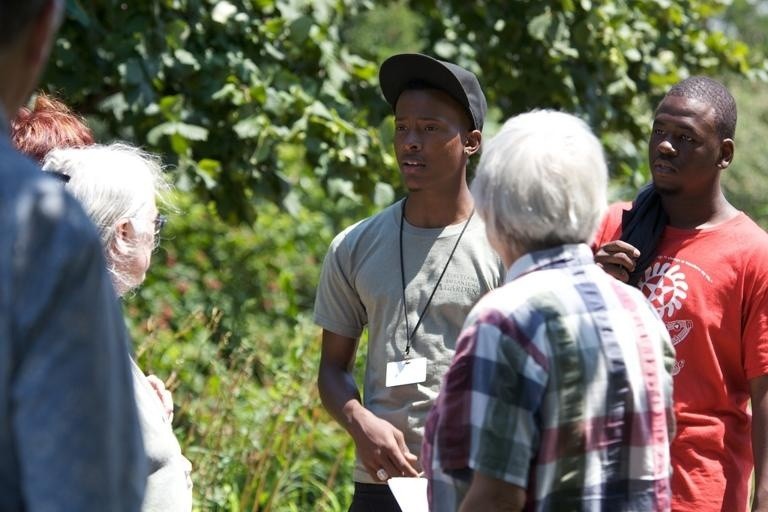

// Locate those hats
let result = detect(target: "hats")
[379,52,487,131]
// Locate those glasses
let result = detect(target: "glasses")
[133,214,166,235]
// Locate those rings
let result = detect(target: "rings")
[374,468,388,482]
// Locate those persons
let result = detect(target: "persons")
[8,90,96,183]
[1,1,149,511]
[38,144,196,512]
[425,104,678,511]
[311,49,508,511]
[590,75,768,511]
